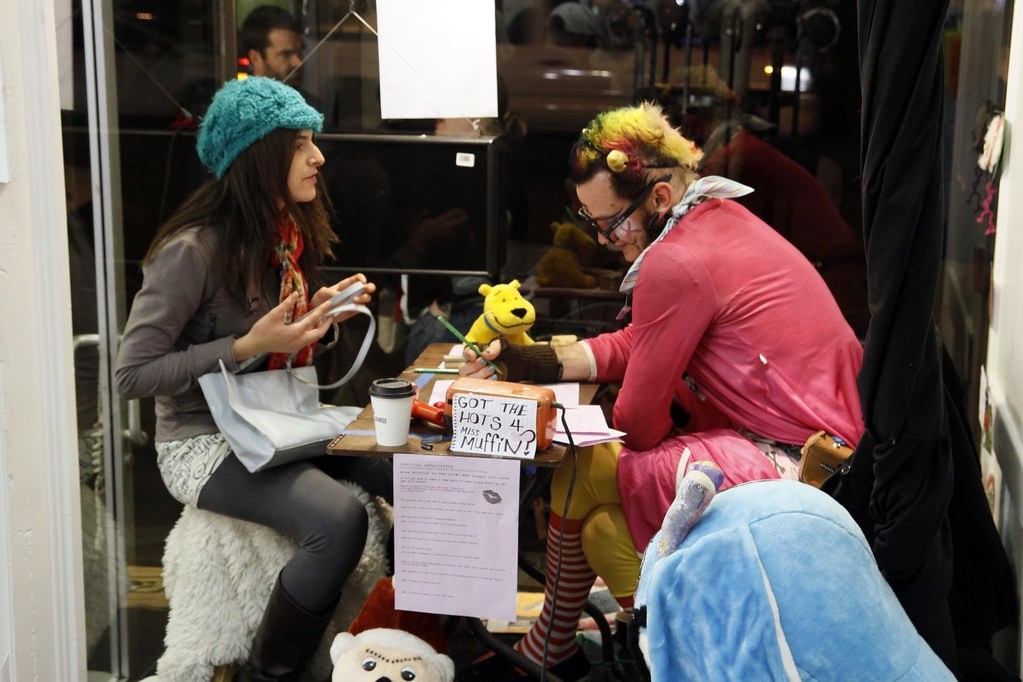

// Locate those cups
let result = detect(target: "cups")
[369,377,416,447]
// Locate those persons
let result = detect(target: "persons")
[115,76,376,682]
[471,101,865,682]
[242,5,337,128]
[658,61,872,340]
[347,76,521,310]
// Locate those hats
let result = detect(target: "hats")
[196,75,324,183]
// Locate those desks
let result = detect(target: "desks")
[332,343,612,682]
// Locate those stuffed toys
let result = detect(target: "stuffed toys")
[632,463,959,682]
[329,628,455,682]
[464,280,537,346]
[536,222,596,305]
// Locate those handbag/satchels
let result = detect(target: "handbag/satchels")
[197,281,375,474]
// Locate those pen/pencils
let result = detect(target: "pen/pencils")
[437,316,503,376]
[414,368,459,374]
[565,207,582,228]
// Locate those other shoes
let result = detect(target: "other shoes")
[462,645,593,682]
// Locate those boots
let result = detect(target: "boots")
[232,565,341,682]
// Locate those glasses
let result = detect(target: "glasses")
[578,174,673,244]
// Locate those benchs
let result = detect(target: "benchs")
[122,443,182,608]
